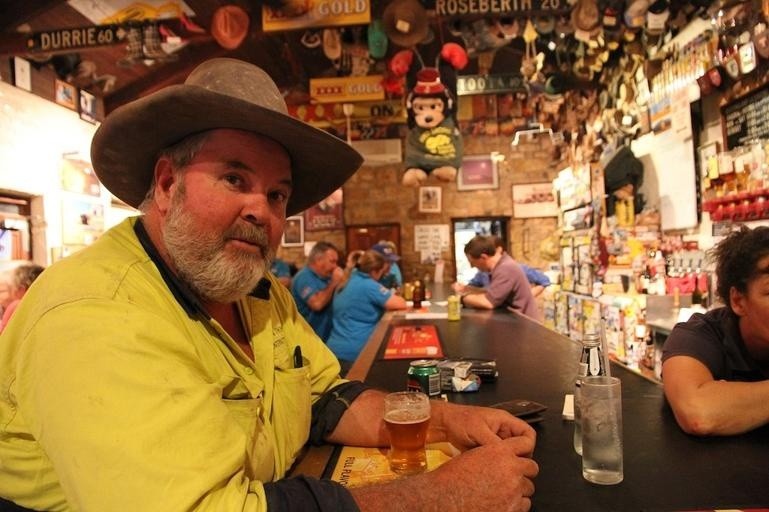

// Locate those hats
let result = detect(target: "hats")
[91,58,363,218]
[373,244,401,261]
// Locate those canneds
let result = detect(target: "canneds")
[447,294,461,321]
[408,360,442,401]
[405,282,412,300]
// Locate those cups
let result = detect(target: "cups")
[579,375,625,486]
[382,390,432,477]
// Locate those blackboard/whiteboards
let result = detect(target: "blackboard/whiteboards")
[723,84,769,151]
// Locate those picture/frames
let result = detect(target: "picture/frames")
[344,223,401,266]
[280,216,304,248]
[13,55,32,91]
[418,184,442,214]
[80,88,97,125]
[512,181,558,220]
[304,187,344,232]
[457,153,500,191]
[54,78,75,109]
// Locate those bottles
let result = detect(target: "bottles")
[411,280,425,309]
[643,264,651,294]
[573,332,609,457]
[651,29,717,105]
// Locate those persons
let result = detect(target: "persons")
[372,239,404,289]
[450,235,540,323]
[267,256,297,288]
[290,241,345,345]
[661,225,769,438]
[1,55,541,510]
[466,236,550,299]
[1,259,46,333]
[324,249,406,379]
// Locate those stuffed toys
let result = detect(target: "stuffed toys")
[259,0,769,145]
[397,66,462,185]
